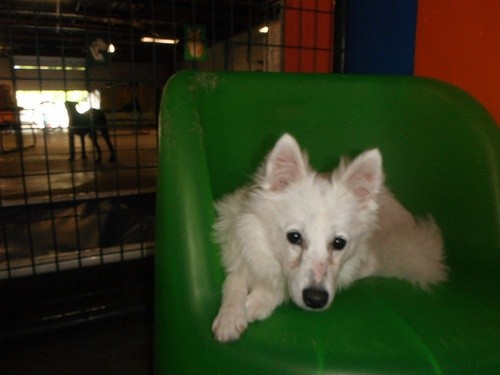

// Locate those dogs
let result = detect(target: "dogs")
[64,101,115,164]
[205,132,451,344]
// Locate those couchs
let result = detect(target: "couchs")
[152,67,499,375]
